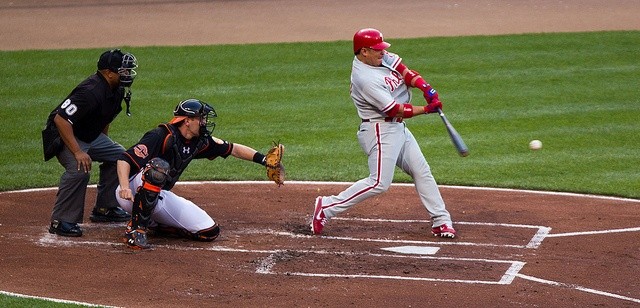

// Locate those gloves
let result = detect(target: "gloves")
[424,99,443,114]
[421,83,439,103]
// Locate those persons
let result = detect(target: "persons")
[115,99,267,250]
[310,28,457,238]
[42,48,138,237]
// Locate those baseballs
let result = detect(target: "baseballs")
[529,139,543,151]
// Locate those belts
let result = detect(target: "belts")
[362,116,403,123]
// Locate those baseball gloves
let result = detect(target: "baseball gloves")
[266,139,286,187]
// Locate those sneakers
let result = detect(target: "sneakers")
[49,217,82,236]
[89,204,131,222]
[310,195,328,234]
[431,223,457,238]
[123,227,150,250]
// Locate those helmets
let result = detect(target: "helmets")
[98,47,138,116]
[353,27,391,54]
[168,98,217,145]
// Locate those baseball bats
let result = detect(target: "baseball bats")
[429,97,470,157]
